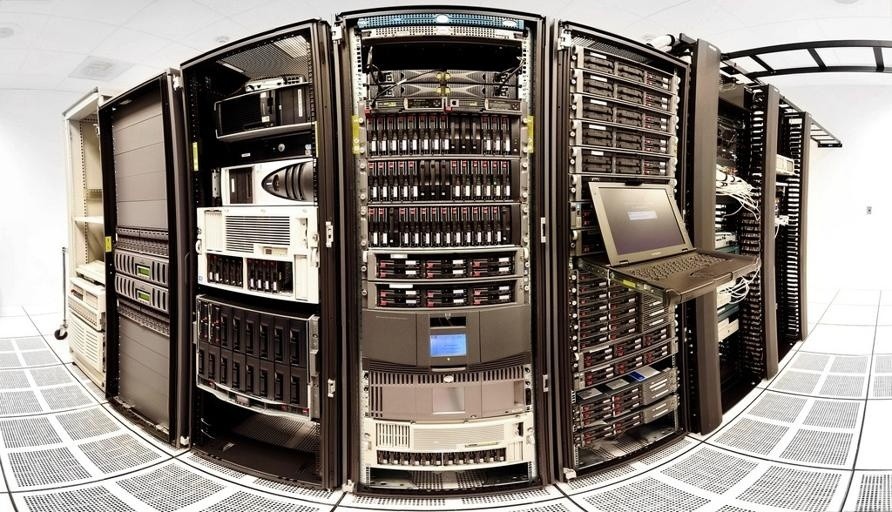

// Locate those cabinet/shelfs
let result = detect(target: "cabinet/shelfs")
[60,6,812,497]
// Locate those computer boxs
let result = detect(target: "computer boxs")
[211,81,315,141]
[215,154,316,206]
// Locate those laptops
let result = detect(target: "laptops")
[588,180,728,282]
